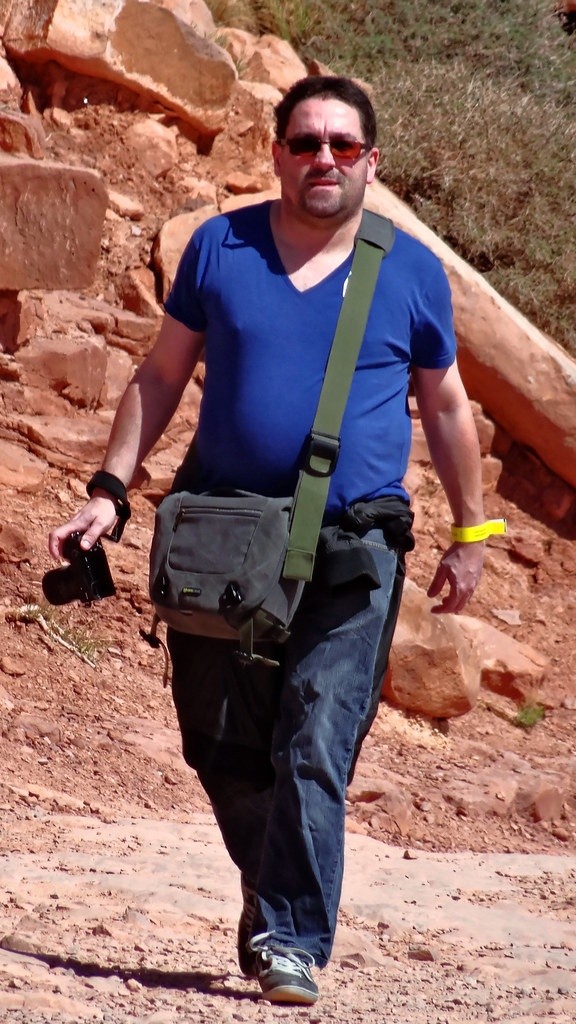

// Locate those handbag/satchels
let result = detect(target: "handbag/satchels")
[148,483,308,642]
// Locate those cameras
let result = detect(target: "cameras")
[42,531,115,605]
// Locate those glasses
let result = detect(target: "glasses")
[275,134,372,159]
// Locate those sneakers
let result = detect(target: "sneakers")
[246,930,319,1004]
[237,871,270,979]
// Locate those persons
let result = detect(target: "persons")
[49,76,508,1006]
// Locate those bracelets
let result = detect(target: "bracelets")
[452,518,506,543]
[85,470,134,516]
